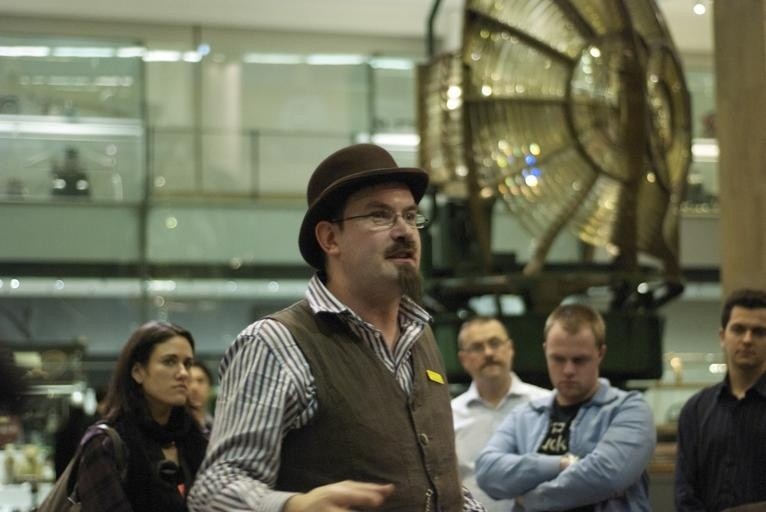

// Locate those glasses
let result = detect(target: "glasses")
[333,210,428,229]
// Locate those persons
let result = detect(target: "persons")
[53,388,95,480]
[450,314,554,512]
[671,288,766,512]
[187,142,467,512]
[186,360,213,440]
[74,318,210,512]
[474,301,658,512]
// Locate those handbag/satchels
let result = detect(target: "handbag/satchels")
[37,424,121,512]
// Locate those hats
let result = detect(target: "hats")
[299,144,428,270]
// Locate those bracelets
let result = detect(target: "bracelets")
[565,450,575,466]
